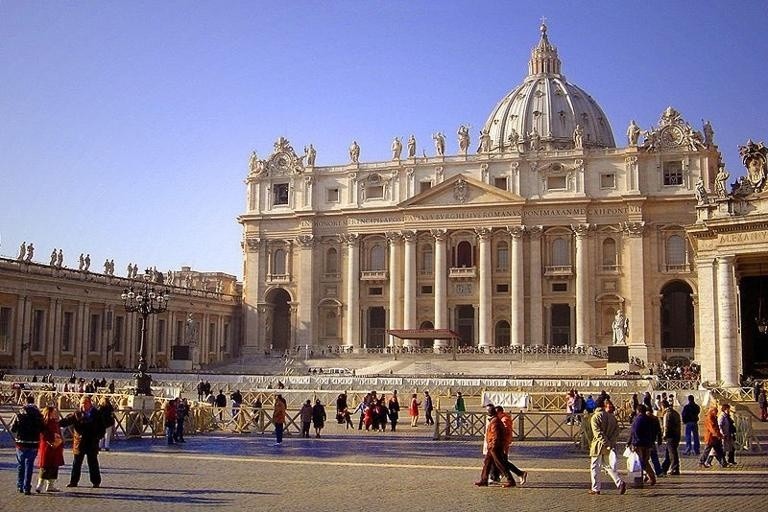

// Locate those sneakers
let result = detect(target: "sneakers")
[588,468,680,495]
[698,457,738,468]
[474,469,527,487]
[17,482,99,495]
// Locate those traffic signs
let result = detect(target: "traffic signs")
[120,266,170,397]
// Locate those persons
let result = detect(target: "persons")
[391,137,402,159]
[529,128,540,151]
[565,355,768,495]
[336,389,400,432]
[488,406,527,486]
[508,128,519,152]
[164,378,287,446]
[573,124,584,148]
[453,392,466,430]
[474,404,517,488]
[714,166,730,199]
[626,120,640,144]
[18,240,224,293]
[300,399,313,438]
[409,394,422,427]
[432,133,445,155]
[694,176,706,205]
[407,135,415,157]
[611,309,629,346]
[351,141,360,162]
[458,125,469,151]
[479,129,490,152]
[312,399,327,438]
[306,144,316,166]
[0,369,115,495]
[423,391,434,426]
[703,120,714,145]
